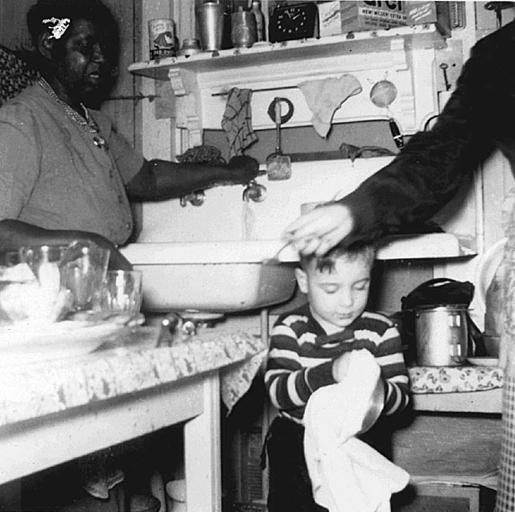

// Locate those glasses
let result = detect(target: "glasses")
[83,124,106,148]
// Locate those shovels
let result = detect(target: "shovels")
[266,96,292,181]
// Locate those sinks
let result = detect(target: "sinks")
[117,240,296,318]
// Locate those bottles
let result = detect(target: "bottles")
[225,1,264,50]
[180,38,200,56]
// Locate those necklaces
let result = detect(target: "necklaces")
[42,79,107,149]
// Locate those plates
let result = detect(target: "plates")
[2,322,121,358]
[467,355,500,365]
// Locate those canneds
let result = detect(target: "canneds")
[148,18,176,59]
[231,11,257,48]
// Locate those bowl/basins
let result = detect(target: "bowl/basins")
[0,244,111,317]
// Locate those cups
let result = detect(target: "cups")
[88,267,143,318]
[196,2,224,51]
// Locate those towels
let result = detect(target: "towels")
[303,348,410,512]
[221,88,258,159]
[296,75,362,137]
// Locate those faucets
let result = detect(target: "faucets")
[179,188,205,207]
[243,177,267,203]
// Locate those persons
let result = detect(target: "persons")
[263,203,410,512]
[283,20,514,510]
[1,1,258,273]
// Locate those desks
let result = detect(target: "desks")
[0,325,271,511]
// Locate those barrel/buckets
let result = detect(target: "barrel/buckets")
[415,304,469,367]
